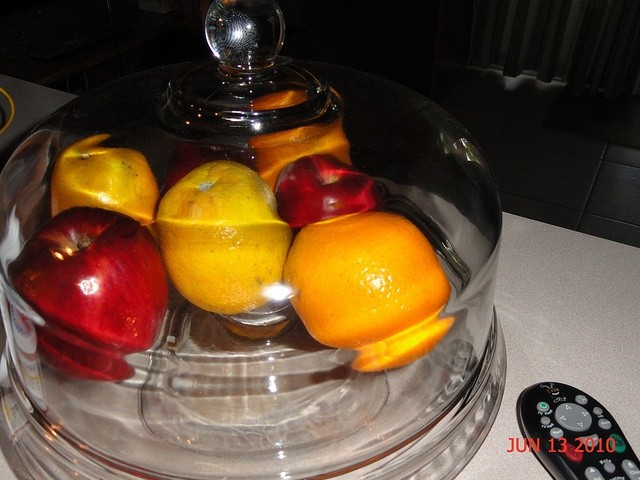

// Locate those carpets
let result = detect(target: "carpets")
[535,79,640,149]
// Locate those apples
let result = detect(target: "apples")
[274,152,378,231]
[9,206,170,384]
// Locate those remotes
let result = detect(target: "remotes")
[517,379,640,480]
